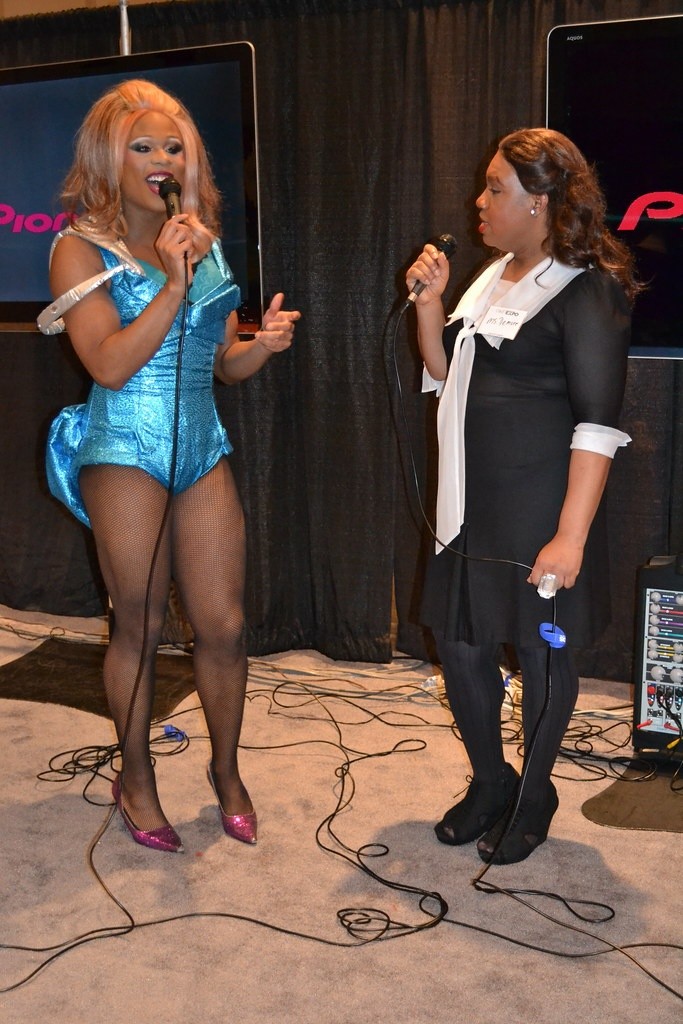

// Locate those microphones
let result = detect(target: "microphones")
[400,235,457,314]
[159,177,189,259]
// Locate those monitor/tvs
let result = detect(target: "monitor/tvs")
[0,41,265,334]
[546,14,683,360]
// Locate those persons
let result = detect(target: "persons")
[35,79,304,856]
[404,128,644,865]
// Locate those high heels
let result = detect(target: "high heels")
[478,776,560,864]
[111,772,184,853]
[433,762,521,846]
[206,764,259,844]
